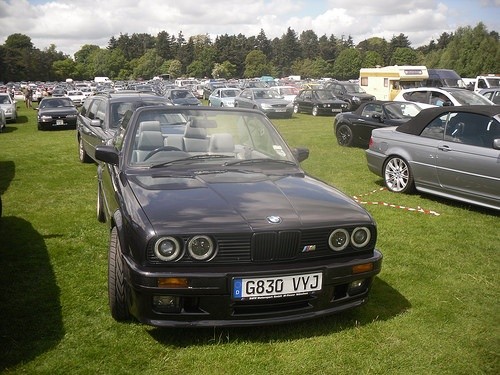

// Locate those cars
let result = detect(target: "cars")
[332,85,500,210]
[77,92,196,165]
[0,77,377,130]
[94,105,383,332]
[460,73,500,107]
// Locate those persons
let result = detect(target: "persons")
[21,87,49,108]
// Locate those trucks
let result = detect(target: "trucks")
[358,65,469,102]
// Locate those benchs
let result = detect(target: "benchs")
[420,118,459,140]
[136,120,210,152]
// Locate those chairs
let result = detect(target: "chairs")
[209,134,239,161]
[455,123,482,146]
[131,131,168,164]
